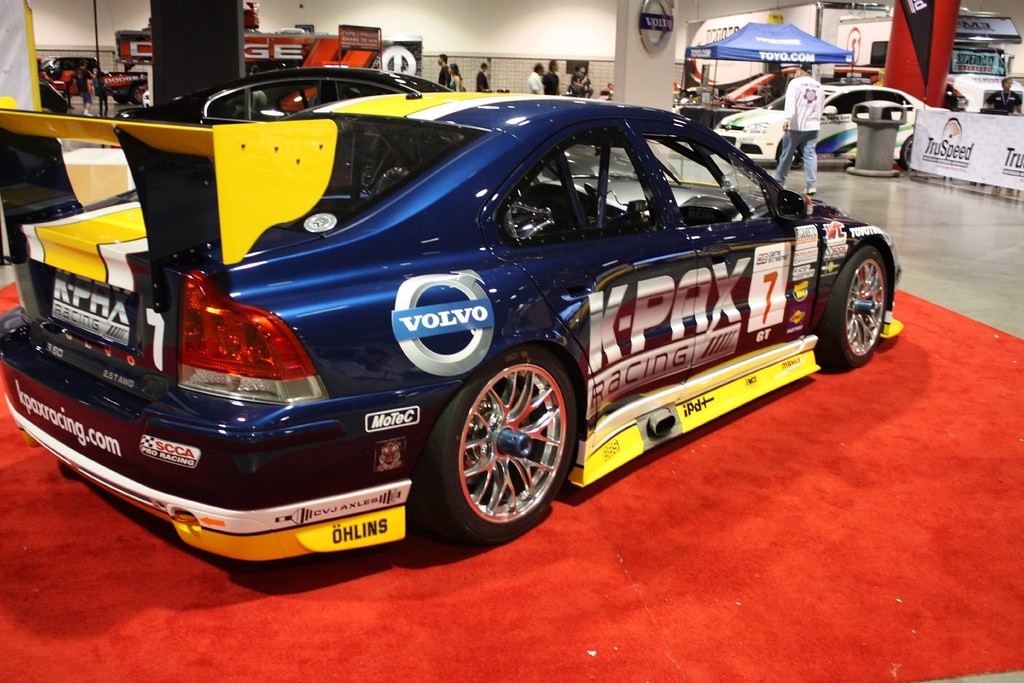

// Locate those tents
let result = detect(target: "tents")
[678,22,854,111]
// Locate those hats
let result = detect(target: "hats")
[794,63,812,74]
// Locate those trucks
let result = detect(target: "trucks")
[41,56,147,105]
[684,2,1023,112]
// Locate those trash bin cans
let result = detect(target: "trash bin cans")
[845,99,908,178]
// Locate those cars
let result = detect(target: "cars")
[116,67,461,206]
[0,93,903,563]
[675,68,967,169]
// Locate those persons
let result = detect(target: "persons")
[527,63,545,94]
[437,54,462,92]
[542,62,559,95]
[73,59,108,118]
[983,77,1022,113]
[604,83,614,92]
[572,65,594,98]
[477,63,492,92]
[771,62,825,195]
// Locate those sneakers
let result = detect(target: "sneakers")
[802,187,817,194]
